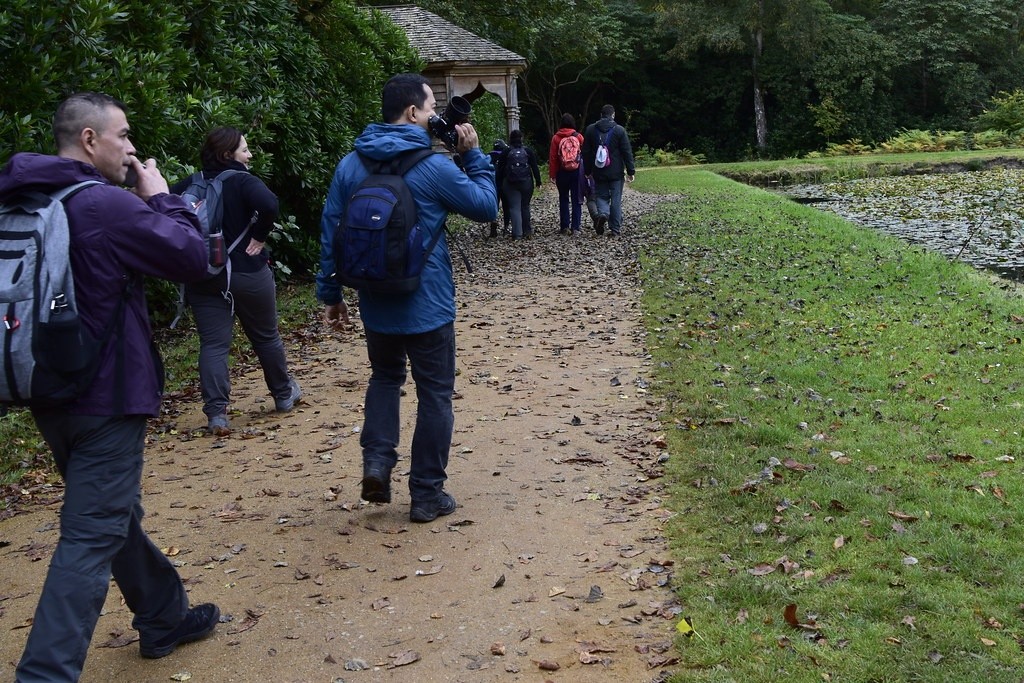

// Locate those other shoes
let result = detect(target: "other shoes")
[612,230,618,235]
[596,214,607,235]
[503,230,510,236]
[571,226,581,236]
[560,228,572,235]
[490,221,497,237]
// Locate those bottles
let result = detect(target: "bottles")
[47,293,77,325]
[210,227,226,267]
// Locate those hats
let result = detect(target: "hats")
[493,139,508,148]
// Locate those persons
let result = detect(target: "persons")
[495,130,541,240]
[487,138,512,236]
[581,104,635,235]
[315,74,497,521]
[184,127,301,427]
[0,93,219,683]
[549,112,584,235]
[579,156,598,227]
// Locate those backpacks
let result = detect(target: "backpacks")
[0,179,107,407]
[493,159,499,179]
[556,131,583,170]
[593,125,616,169]
[184,169,259,275]
[507,144,531,180]
[332,145,446,309]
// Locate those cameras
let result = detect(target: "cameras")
[428,95,471,149]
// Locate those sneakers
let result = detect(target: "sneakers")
[410,490,457,522]
[275,379,300,411]
[207,413,228,432]
[141,602,219,659]
[357,457,391,505]
